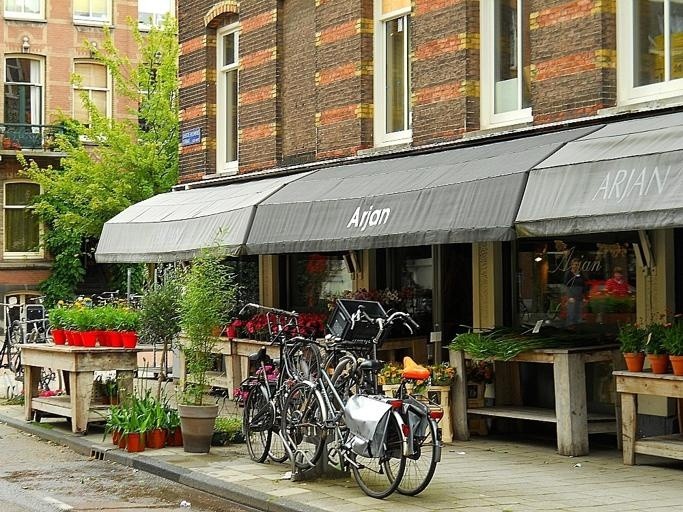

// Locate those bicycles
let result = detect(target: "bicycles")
[239,299,444,499]
[1,288,143,372]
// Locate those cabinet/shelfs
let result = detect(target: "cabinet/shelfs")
[177,332,453,443]
[12,343,144,434]
[610,368,683,466]
[448,342,630,457]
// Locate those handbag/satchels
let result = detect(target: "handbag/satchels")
[344,394,393,458]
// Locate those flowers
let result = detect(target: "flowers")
[233,311,328,336]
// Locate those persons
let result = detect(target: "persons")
[604,268,633,298]
[563,258,589,288]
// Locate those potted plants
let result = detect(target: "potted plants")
[172,226,245,454]
[615,316,683,375]
[88,386,183,454]
[51,306,138,348]
